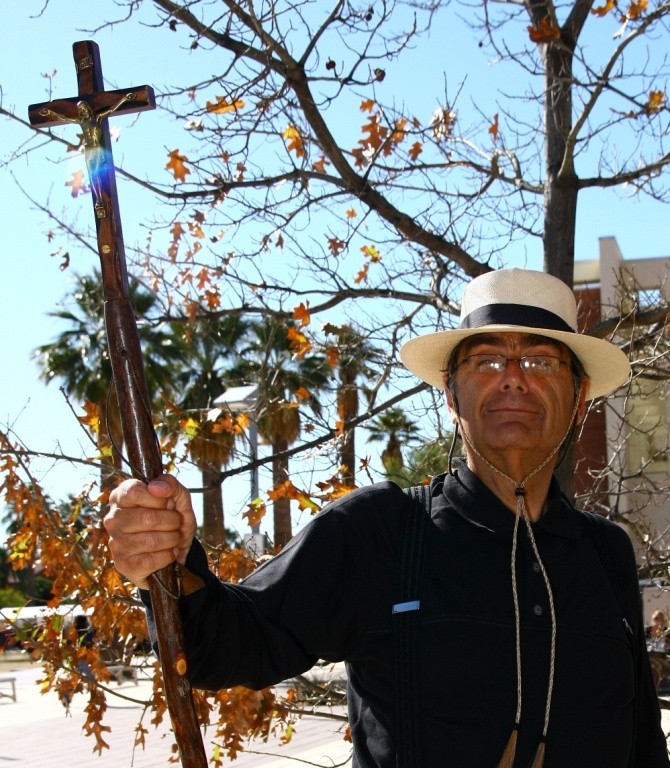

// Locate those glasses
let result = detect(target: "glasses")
[453,354,568,375]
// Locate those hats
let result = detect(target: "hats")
[400,268,630,401]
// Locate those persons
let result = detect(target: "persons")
[100,268,670,768]
[643,611,670,693]
